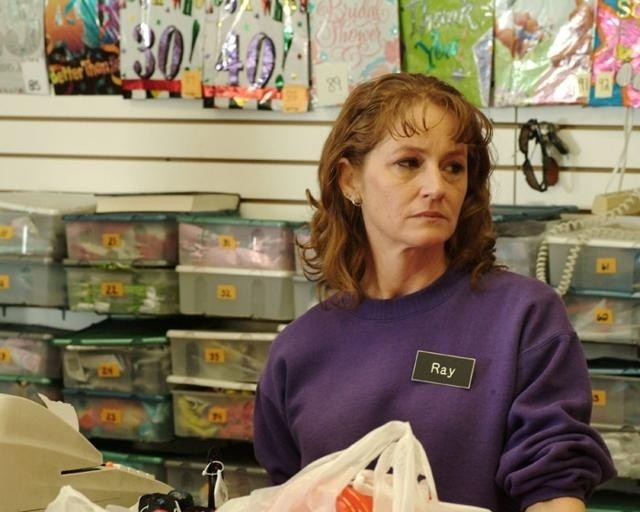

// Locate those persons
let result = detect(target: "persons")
[249,71,619,511]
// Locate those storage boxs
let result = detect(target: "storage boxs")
[1,194,640,508]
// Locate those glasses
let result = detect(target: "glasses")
[519,119,567,192]
[150,490,191,511]
